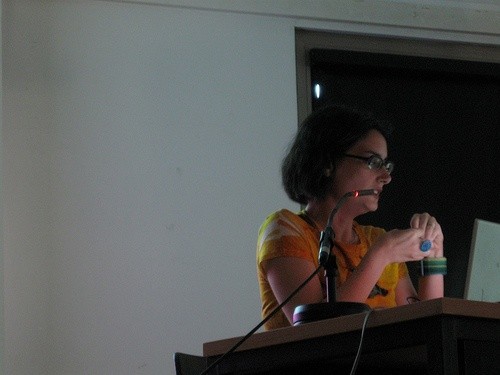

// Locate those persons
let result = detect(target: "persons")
[256,95,447,332]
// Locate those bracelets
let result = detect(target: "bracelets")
[420,257,447,277]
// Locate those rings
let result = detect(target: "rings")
[420,236,430,251]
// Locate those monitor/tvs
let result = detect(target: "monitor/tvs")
[462,218,500,303]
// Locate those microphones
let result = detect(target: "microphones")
[318,189,378,266]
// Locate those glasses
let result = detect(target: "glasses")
[336,154,394,177]
